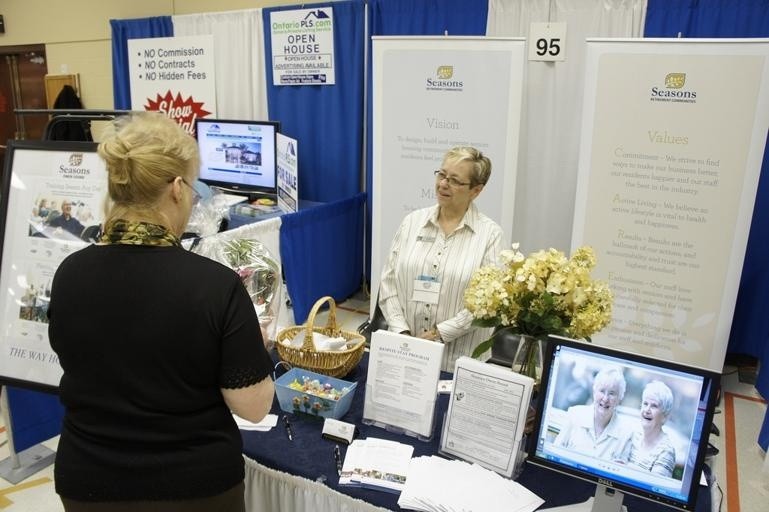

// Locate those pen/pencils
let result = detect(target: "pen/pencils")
[283,416,292,440]
[334,444,342,476]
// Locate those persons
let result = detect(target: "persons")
[555,365,626,462]
[615,379,678,478]
[376,146,508,373]
[45,110,275,512]
[35,197,86,239]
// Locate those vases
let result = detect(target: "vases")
[504,337,548,385]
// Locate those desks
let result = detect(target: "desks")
[231,343,720,512]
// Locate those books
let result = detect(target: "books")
[338,435,545,511]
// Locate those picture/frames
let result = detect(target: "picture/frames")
[0,139,111,393]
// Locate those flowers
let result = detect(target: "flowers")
[460,239,613,360]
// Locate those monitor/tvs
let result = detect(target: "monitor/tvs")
[524,334,722,512]
[194,119,279,205]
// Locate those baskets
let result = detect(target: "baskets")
[276,296,366,379]
[273,361,359,420]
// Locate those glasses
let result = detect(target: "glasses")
[166,176,202,205]
[435,170,484,187]
